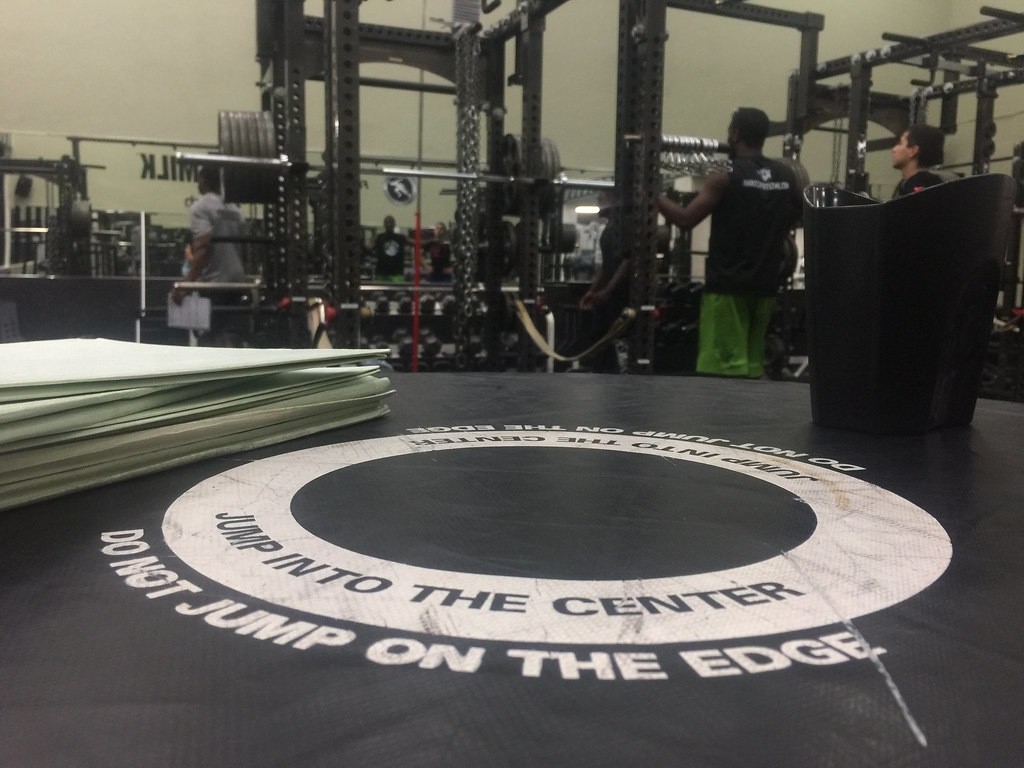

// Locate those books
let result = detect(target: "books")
[0,337,397,514]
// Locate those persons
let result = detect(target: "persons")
[655,107,800,380]
[361,214,414,283]
[172,162,250,346]
[574,184,634,374]
[419,222,453,283]
[890,125,945,196]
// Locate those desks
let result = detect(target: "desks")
[0,371,1024,768]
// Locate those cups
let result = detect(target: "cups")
[801,172,1014,437]
